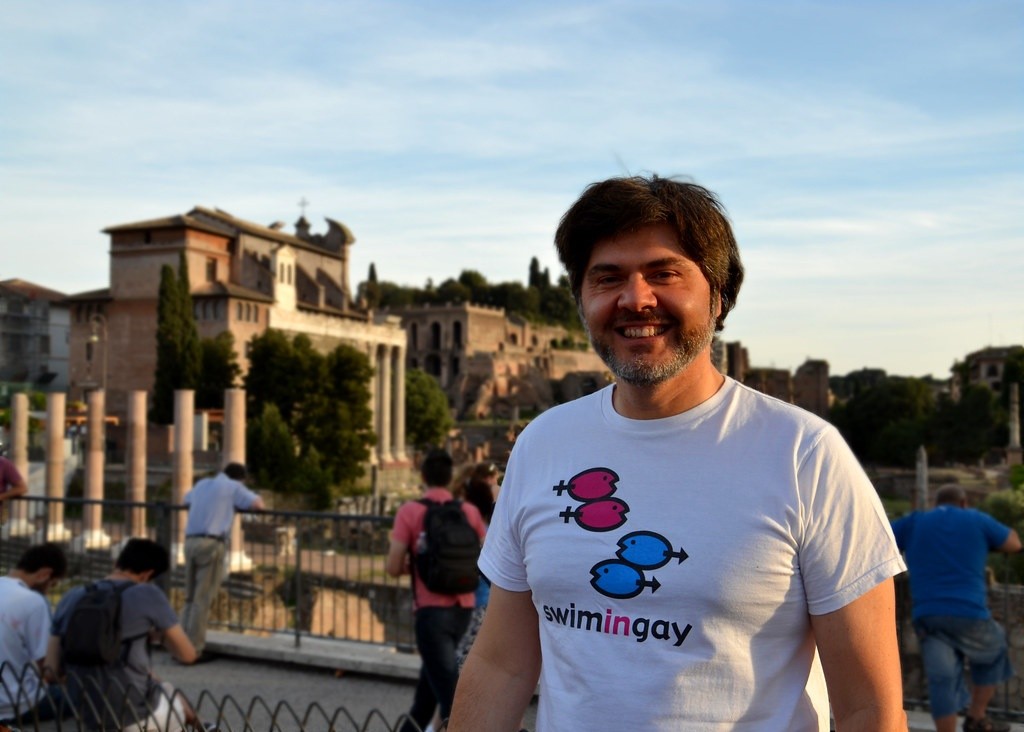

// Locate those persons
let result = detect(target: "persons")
[891,485,1021,731]
[448,175,912,732]
[0,543,79,726]
[454,462,504,608]
[171,462,263,665]
[0,449,28,504]
[44,538,206,732]
[387,449,489,732]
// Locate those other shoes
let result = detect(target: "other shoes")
[193,649,221,664]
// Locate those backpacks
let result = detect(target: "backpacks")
[407,497,482,596]
[61,579,134,669]
